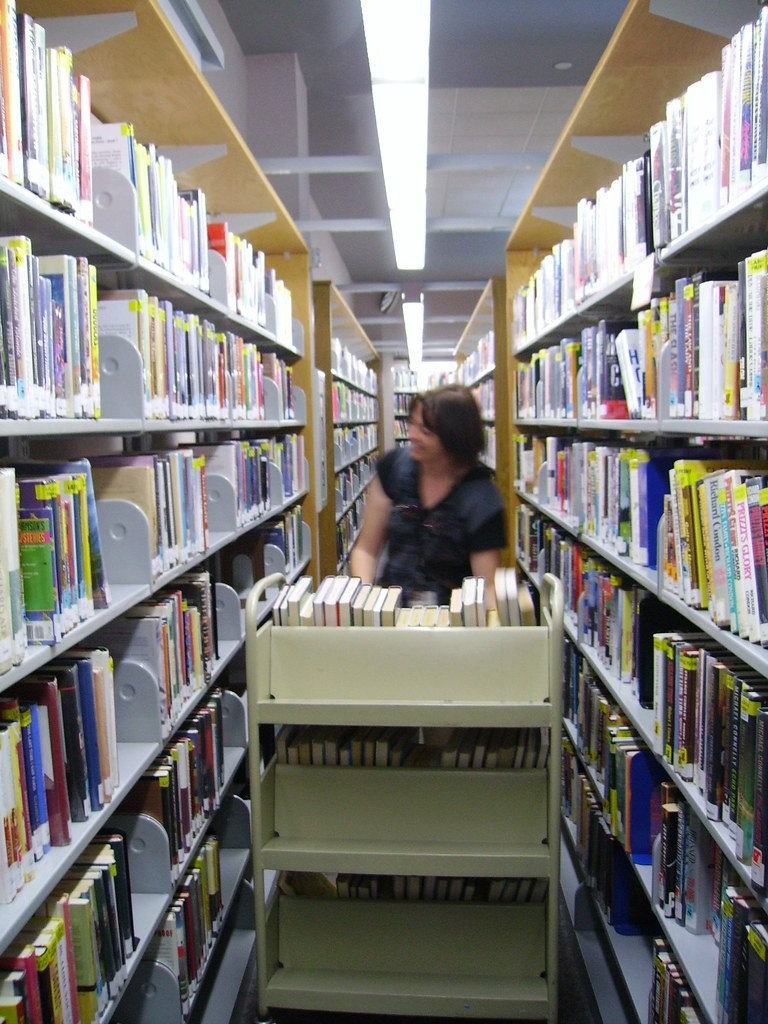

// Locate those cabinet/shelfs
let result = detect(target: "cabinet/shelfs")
[0,0,324,1024]
[246,572,566,1024]
[312,277,384,595]
[500,0,768,1024]
[452,274,510,568]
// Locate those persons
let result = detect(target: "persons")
[352,384,509,746]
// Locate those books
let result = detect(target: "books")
[0,11,768,1024]
